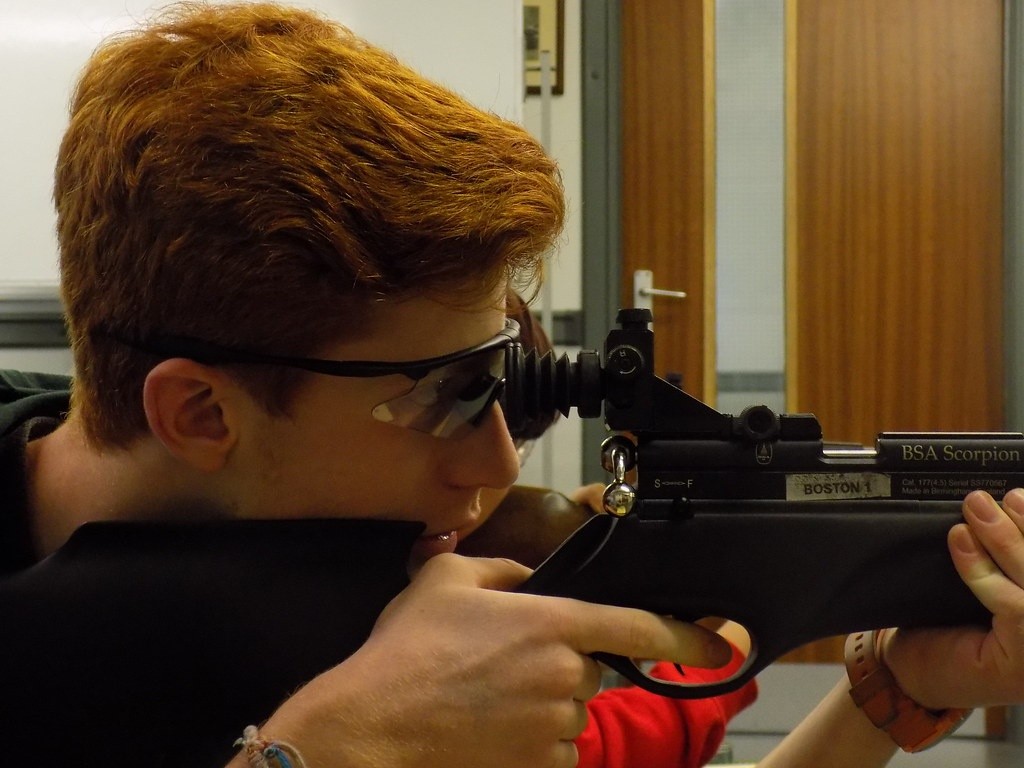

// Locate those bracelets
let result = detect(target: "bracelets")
[846,630,974,753]
[235,725,304,768]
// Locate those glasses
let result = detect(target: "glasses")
[115,317,521,441]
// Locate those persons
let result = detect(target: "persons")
[0,0,1024,768]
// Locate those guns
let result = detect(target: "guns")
[0,307,1024,768]
